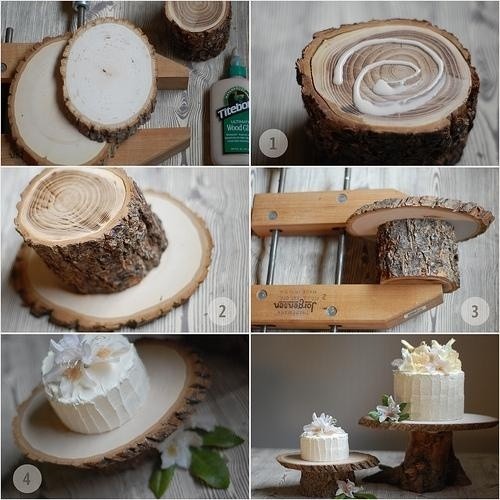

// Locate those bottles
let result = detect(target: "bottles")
[208,47,249,166]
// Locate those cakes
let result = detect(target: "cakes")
[299,411,349,462]
[41,334,150,434]
[390,336,464,421]
